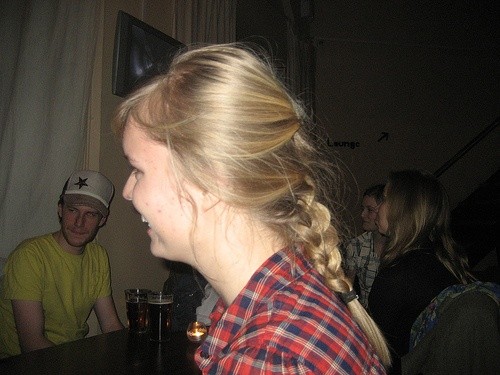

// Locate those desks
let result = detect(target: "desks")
[0,327,203,375]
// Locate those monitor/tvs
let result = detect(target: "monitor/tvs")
[112,10,188,97]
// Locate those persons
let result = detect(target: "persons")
[0,169,127,358]
[112,43,391,375]
[342,170,500,375]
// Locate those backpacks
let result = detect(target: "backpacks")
[162,262,208,333]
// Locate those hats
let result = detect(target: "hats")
[61,171,115,219]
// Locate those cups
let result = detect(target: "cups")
[124,288,152,333]
[147,292,173,342]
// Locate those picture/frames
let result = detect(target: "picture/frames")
[112,10,186,98]
